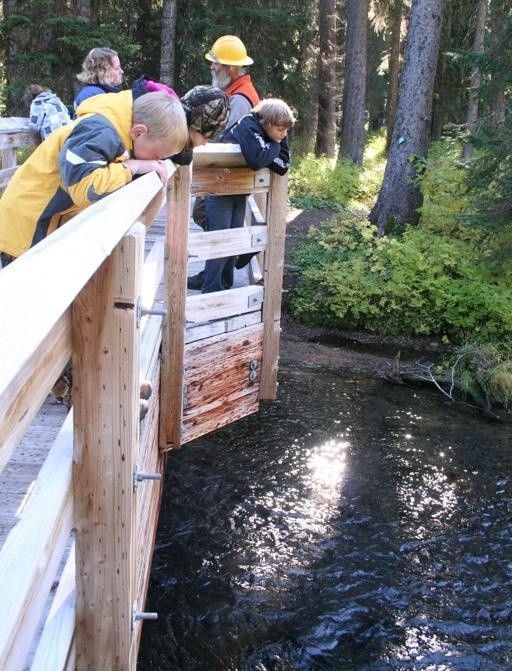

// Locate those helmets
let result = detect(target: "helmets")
[205,36,254,66]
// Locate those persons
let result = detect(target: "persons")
[22,84,72,141]
[71,48,123,116]
[0,90,190,419]
[200,98,295,294]
[130,75,231,164]
[186,36,260,289]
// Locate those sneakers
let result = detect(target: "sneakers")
[235,254,259,270]
[187,270,205,290]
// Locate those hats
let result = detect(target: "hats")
[180,84,231,141]
